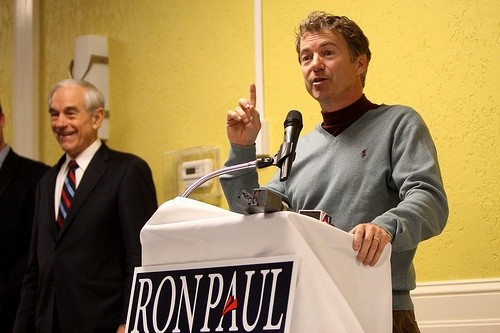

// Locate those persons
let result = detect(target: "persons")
[218,7,450,332]
[0,105,51,332]
[12,77,158,333]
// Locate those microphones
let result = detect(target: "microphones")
[278,109,303,182]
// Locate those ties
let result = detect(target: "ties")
[56,160,79,238]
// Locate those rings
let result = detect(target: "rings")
[374,236,380,243]
